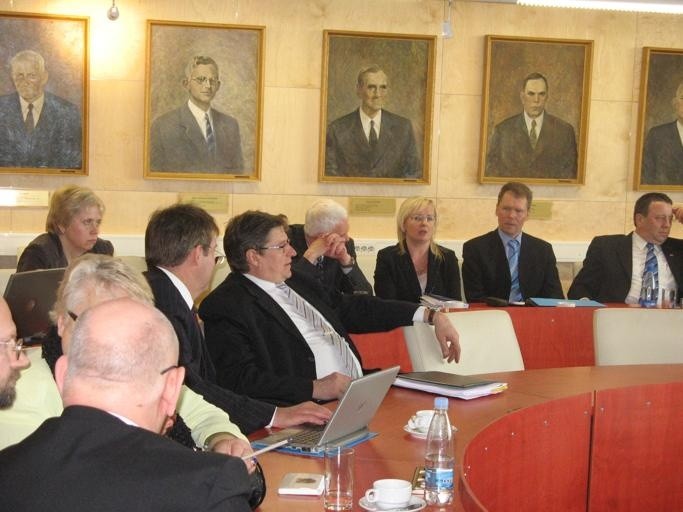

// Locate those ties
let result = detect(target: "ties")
[316,255,326,281]
[203,111,216,164]
[25,103,36,136]
[367,118,380,150]
[639,241,659,308]
[505,239,523,304]
[528,118,541,151]
[275,282,359,380]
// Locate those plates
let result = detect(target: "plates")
[402,425,460,442]
[359,495,426,511]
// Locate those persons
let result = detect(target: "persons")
[0,51,84,169]
[1,183,372,510]
[486,73,577,180]
[462,182,565,303]
[324,62,422,179]
[567,193,683,304]
[150,55,245,175]
[641,82,683,186]
[372,194,462,301]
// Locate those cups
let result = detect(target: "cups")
[445,300,465,309]
[364,478,412,508]
[556,301,576,307]
[412,409,435,434]
[662,287,677,309]
[323,446,354,510]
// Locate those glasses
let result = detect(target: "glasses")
[207,246,225,267]
[255,240,289,251]
[409,214,434,223]
[189,75,221,85]
[0,337,24,360]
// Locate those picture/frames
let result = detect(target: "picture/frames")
[630,47,683,194]
[143,19,265,182]
[0,11,92,178]
[318,27,436,186]
[477,34,596,188]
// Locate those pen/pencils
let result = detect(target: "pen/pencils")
[241,438,293,459]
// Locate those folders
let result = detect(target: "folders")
[527,298,608,307]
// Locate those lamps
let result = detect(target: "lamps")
[106,0,119,19]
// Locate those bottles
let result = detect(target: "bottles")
[423,396,455,508]
[639,262,658,307]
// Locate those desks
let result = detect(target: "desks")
[243,364,683,510]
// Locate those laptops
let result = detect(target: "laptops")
[3,268,64,346]
[256,365,401,453]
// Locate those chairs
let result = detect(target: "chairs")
[593,307,682,367]
[401,309,527,374]
